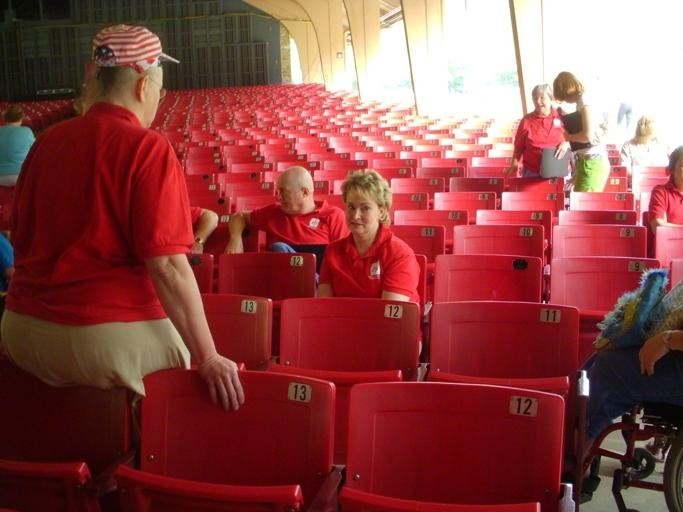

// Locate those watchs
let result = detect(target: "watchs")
[194,236,206,247]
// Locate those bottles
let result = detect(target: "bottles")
[559,483,575,512]
[579,370,590,396]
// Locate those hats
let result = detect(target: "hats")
[92,24,180,73]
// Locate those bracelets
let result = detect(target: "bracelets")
[662,330,673,352]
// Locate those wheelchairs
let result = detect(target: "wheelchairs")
[581,401,682,512]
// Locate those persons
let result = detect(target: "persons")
[222,166,348,274]
[318,169,422,356]
[504,85,570,192]
[0,232,15,297]
[188,207,219,244]
[581,283,683,458]
[0,106,37,187]
[552,71,612,193]
[620,117,668,176]
[0,23,245,410]
[648,146,683,229]
